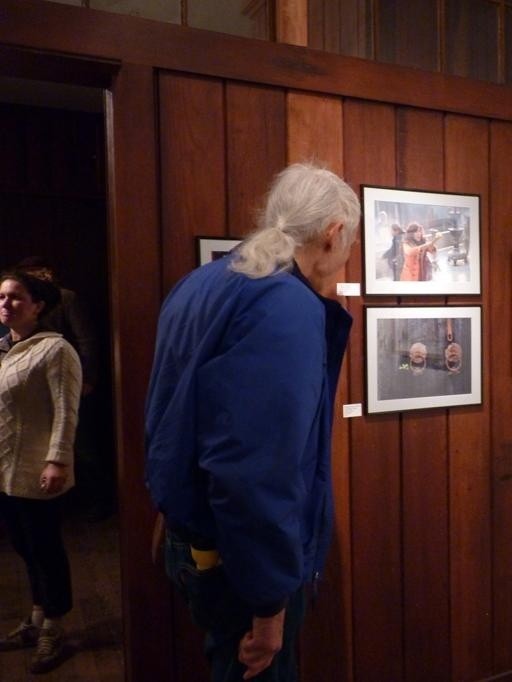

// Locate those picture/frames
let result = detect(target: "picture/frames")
[195,237,243,270]
[362,305,482,415]
[361,182,483,300]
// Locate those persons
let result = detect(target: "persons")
[399,222,438,282]
[380,222,404,281]
[141,153,364,681]
[0,264,84,677]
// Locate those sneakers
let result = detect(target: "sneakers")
[29,620,67,675]
[0,614,41,652]
